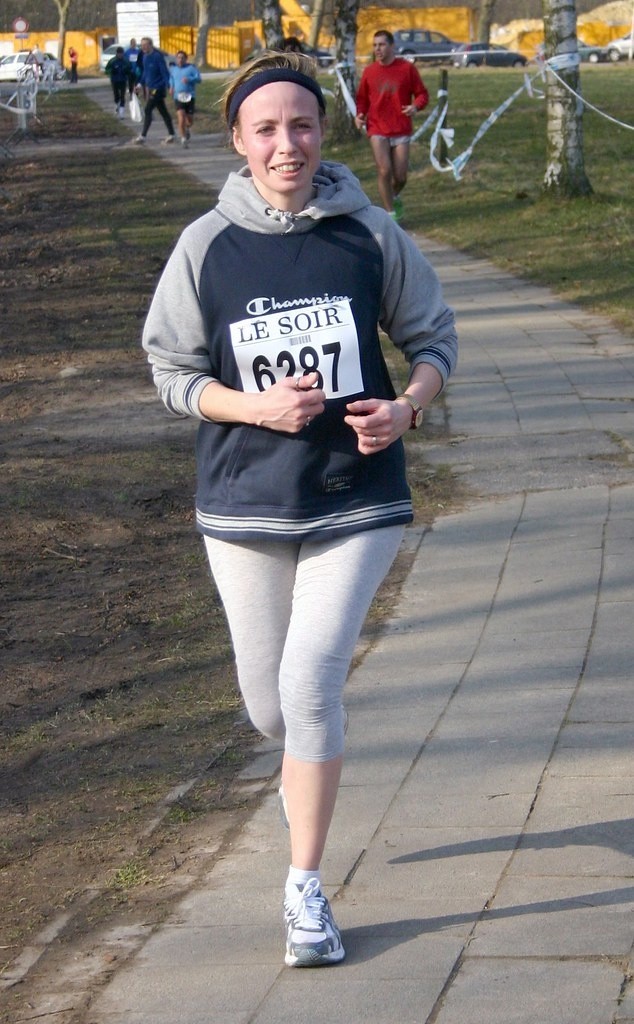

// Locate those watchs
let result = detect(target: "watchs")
[396,394,423,430]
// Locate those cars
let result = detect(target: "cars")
[299,41,333,66]
[606,33,634,62]
[391,28,467,63]
[101,44,177,72]
[0,52,64,80]
[448,43,528,70]
[536,39,610,63]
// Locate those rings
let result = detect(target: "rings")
[372,436,376,446]
[306,416,310,425]
[296,377,300,390]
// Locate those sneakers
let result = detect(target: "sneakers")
[392,196,404,220]
[390,212,397,223]
[283,878,345,967]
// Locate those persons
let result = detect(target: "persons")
[33,45,44,81]
[142,51,457,966]
[355,31,429,224]
[105,37,201,148]
[68,47,78,83]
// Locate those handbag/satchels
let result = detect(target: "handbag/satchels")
[130,93,145,123]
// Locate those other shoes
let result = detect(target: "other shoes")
[182,138,187,148]
[135,136,145,142]
[186,128,191,139]
[164,134,177,143]
